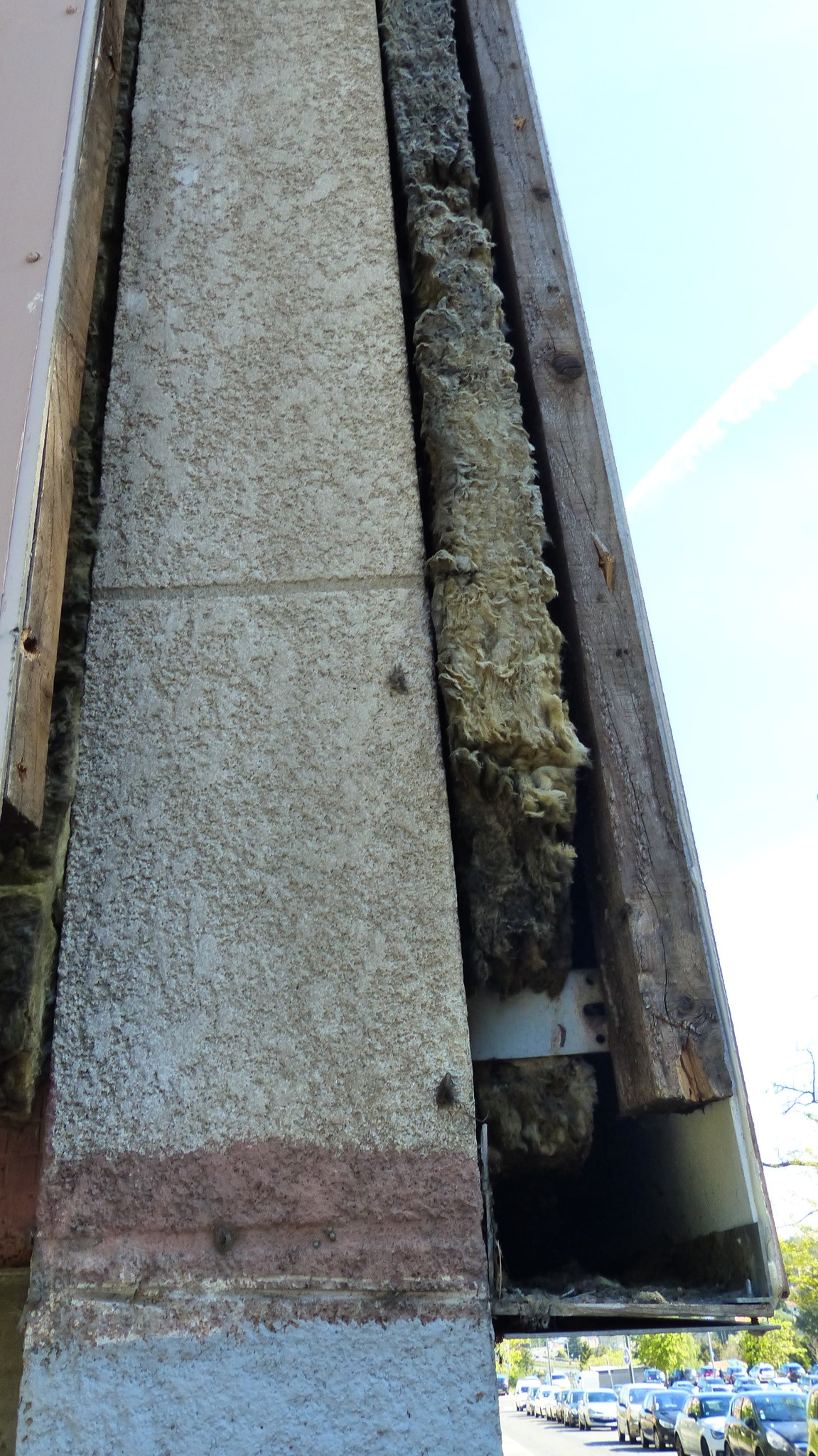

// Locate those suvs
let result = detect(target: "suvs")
[723,1390,809,1456]
[544,1373,571,1389]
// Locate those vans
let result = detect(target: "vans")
[616,1382,668,1444]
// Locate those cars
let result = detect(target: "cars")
[496,1376,510,1395]
[577,1388,619,1431]
[673,1391,739,1456]
[514,1378,573,1424]
[805,1383,818,1456]
[643,1362,818,1391]
[563,1389,588,1428]
[638,1389,696,1451]
